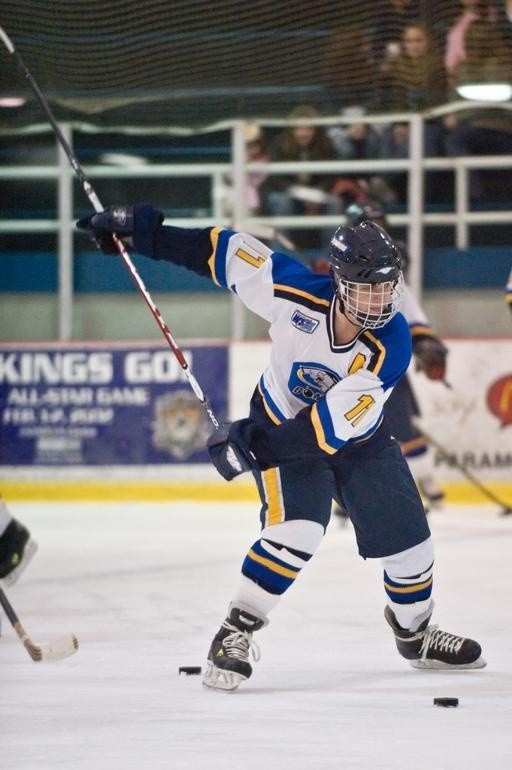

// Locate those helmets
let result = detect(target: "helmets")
[328,220,402,330]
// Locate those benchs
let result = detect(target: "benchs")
[0,0,512,219]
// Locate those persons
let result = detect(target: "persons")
[1,495,38,595]
[225,0,511,249]
[331,202,449,518]
[77,202,483,680]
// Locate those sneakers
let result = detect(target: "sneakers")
[1,517,29,578]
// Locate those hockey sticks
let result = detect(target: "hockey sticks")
[412,419,512,516]
[0,588,78,661]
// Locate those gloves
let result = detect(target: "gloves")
[208,420,267,482]
[77,200,162,261]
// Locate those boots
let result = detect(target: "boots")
[385,598,481,663]
[208,599,270,678]
